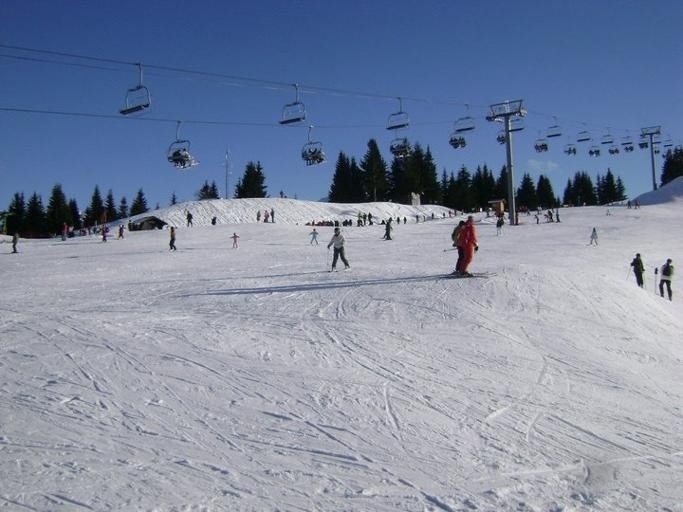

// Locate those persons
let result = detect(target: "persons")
[597,200,600,205]
[264,210,269,222]
[606,208,611,216]
[172,147,196,168]
[590,227,598,244]
[564,143,666,158]
[169,226,177,250]
[270,208,274,223]
[3,230,6,235]
[11,230,19,253]
[631,253,645,287]
[295,205,561,277]
[497,134,507,144]
[230,233,240,248]
[390,144,412,159]
[659,259,674,301]
[535,143,548,152]
[211,216,217,225]
[257,210,261,223]
[187,210,193,228]
[449,136,466,149]
[626,197,640,209]
[51,218,132,243]
[302,147,326,166]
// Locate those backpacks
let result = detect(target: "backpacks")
[664,264,674,275]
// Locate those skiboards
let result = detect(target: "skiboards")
[437,271,497,278]
